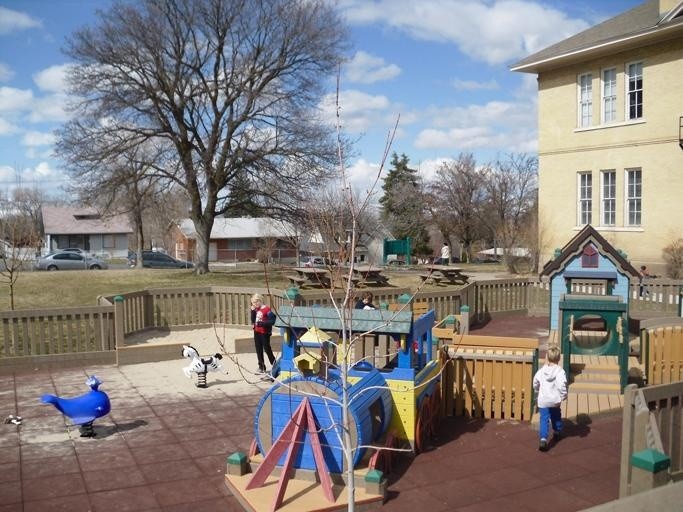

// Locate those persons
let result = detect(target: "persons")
[251,293,276,375]
[638,266,657,296]
[355,291,376,310]
[441,242,450,265]
[533,347,568,451]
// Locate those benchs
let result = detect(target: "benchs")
[343,274,392,288]
[419,274,469,286]
[288,275,336,289]
[387,302,429,323]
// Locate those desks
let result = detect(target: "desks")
[425,265,463,286]
[352,267,384,287]
[291,267,330,290]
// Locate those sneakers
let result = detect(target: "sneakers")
[552,432,562,440]
[538,440,547,451]
[255,368,266,375]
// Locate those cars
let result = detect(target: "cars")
[129,252,195,269]
[297,256,338,268]
[34,248,108,270]
